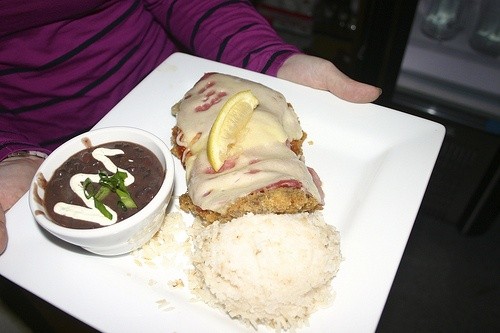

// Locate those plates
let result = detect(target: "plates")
[0,51,447,333]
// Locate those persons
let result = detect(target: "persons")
[0,0,383,258]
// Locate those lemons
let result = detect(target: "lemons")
[207,91,259,172]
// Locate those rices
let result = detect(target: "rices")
[130,207,343,332]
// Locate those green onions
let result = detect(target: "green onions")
[82,169,137,220]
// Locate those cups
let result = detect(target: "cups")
[27,126,176,258]
[416,0,462,41]
